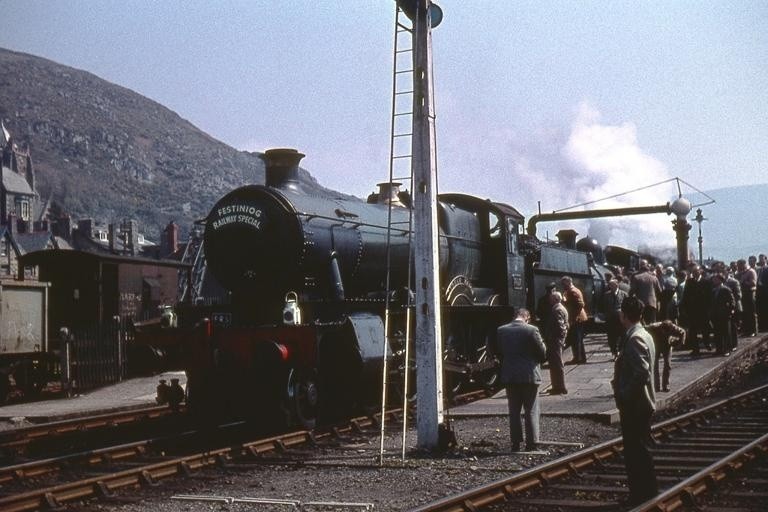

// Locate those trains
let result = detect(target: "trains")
[153,149,722,429]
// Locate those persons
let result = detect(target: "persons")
[560,275,589,365]
[494,307,548,452]
[536,281,557,320]
[544,291,571,395]
[644,319,687,392]
[610,295,658,510]
[600,254,768,361]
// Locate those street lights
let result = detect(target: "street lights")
[671,198,692,275]
